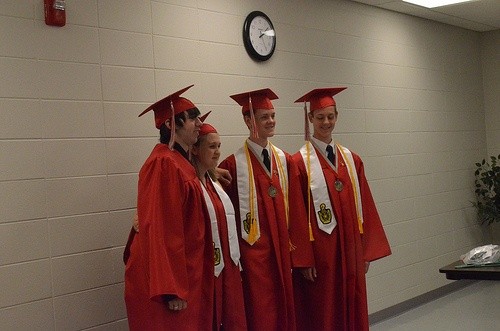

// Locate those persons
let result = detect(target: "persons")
[293,87,393,331]
[192,112,246,330]
[124,85,232,331]
[217,88,313,330]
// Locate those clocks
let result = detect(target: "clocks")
[242,11,276,62]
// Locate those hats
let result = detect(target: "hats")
[138,84,195,150]
[293,87,348,141]
[197,111,217,139]
[229,88,279,138]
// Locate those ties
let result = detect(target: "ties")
[326,146,336,167]
[262,149,270,173]
